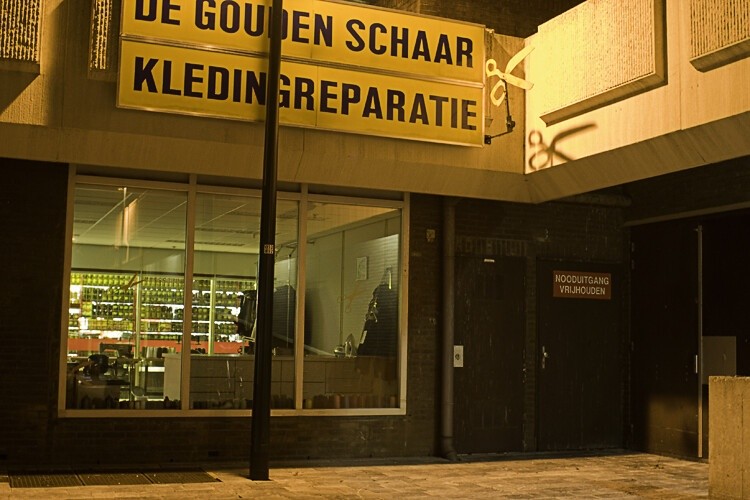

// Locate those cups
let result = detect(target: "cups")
[304,393,396,409]
[193,400,252,409]
[142,346,175,359]
[80,395,180,410]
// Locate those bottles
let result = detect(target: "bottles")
[69,273,256,342]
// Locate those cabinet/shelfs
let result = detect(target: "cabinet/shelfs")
[70,271,376,403]
